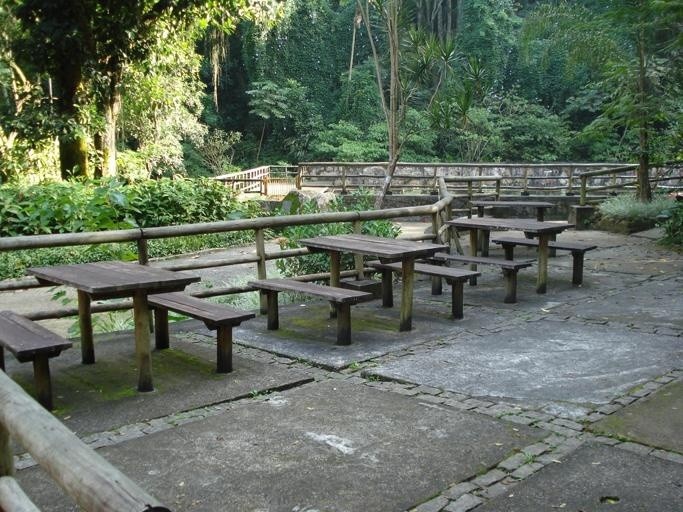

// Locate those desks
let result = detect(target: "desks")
[469,200,556,222]
[26,260,201,393]
[295,233,451,332]
[443,217,576,294]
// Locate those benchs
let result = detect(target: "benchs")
[147,291,256,373]
[434,253,533,304]
[0,310,73,412]
[380,262,481,321]
[491,237,598,286]
[248,278,373,346]
